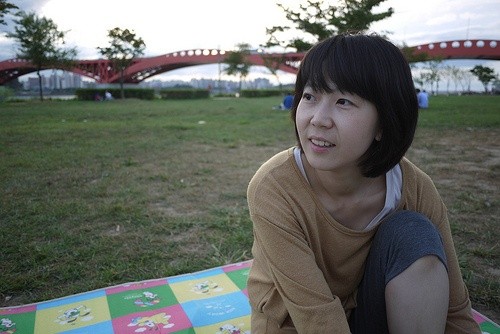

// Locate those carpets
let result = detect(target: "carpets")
[0,259,500,334]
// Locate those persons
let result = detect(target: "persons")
[283,92,292,109]
[246,35,482,334]
[415,89,429,109]
